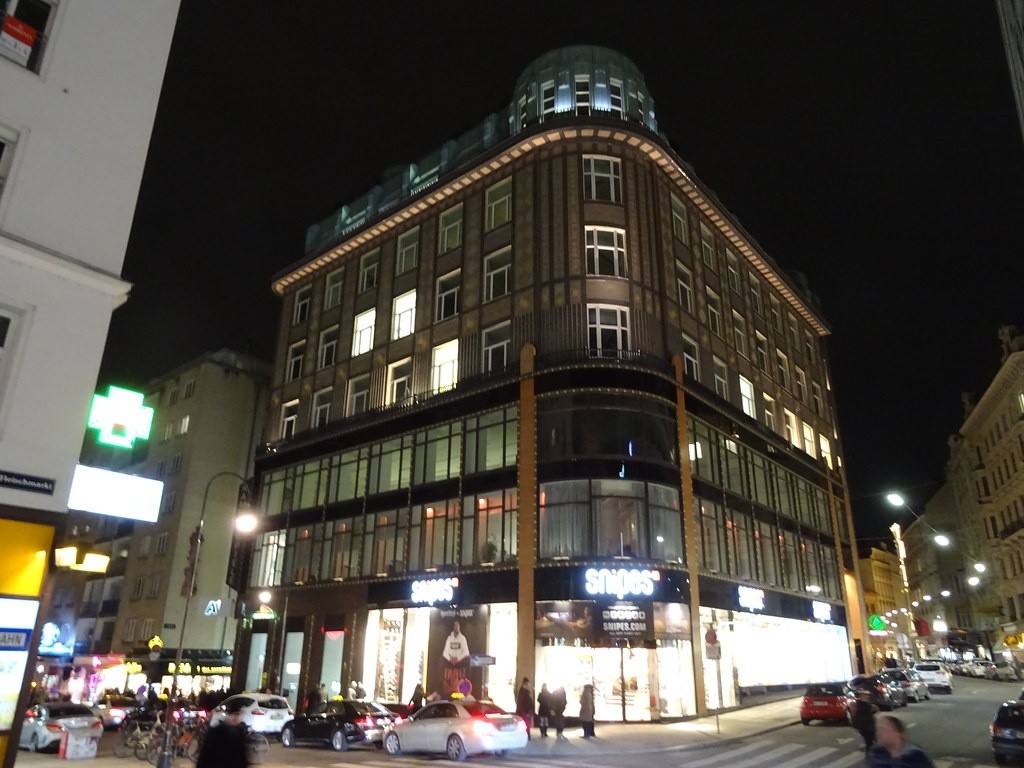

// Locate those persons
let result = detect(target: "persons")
[537,683,552,737]
[552,687,567,735]
[162,685,228,712]
[845,697,879,752]
[195,702,252,768]
[346,681,367,700]
[866,711,936,768]
[408,684,428,714]
[306,683,328,712]
[880,653,898,668]
[516,677,534,740]
[580,684,595,738]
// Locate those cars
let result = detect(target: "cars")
[988,687,1024,765]
[912,658,954,694]
[850,673,908,711]
[799,682,860,725]
[137,699,207,722]
[89,697,137,731]
[878,668,931,703]
[18,703,104,752]
[944,659,1024,682]
[382,698,529,762]
[281,699,402,752]
[210,690,295,742]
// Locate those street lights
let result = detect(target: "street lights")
[54,540,113,703]
[156,471,262,768]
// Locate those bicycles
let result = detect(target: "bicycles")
[111,718,271,767]
